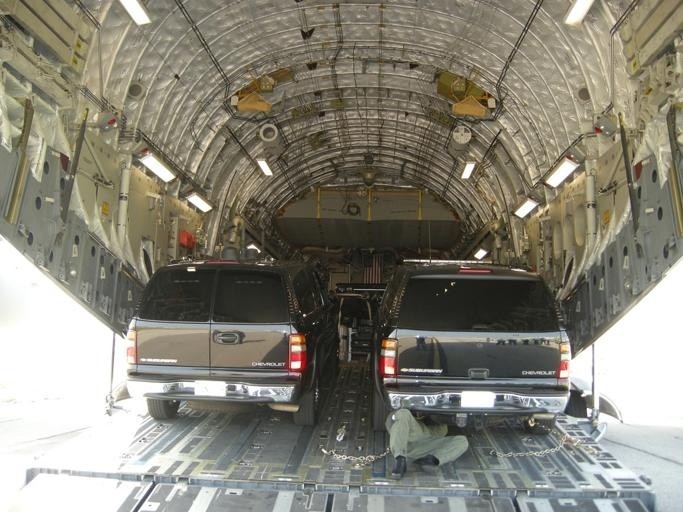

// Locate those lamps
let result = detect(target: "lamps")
[561,0,596,26]
[474,248,487,260]
[139,154,176,183]
[185,192,213,212]
[514,197,539,218]
[119,0,152,25]
[545,156,580,188]
[256,158,274,176]
[461,161,477,179]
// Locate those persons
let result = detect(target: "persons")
[384,407,469,480]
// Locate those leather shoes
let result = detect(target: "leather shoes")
[411,456,440,475]
[391,458,407,479]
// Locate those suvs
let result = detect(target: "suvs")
[121,257,339,429]
[371,255,570,437]
